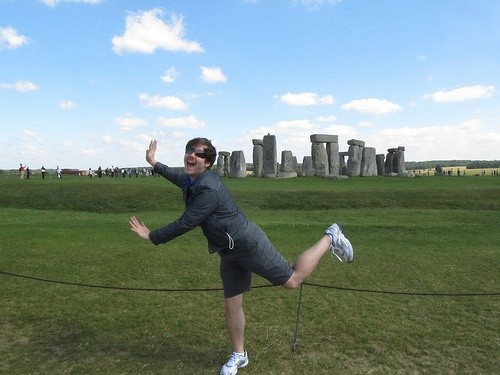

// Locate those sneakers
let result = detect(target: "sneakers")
[323,222,354,263]
[219,349,248,375]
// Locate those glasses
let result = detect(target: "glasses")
[186,147,206,158]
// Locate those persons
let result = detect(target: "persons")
[413,168,499,176]
[18,164,161,179]
[129,138,354,375]
